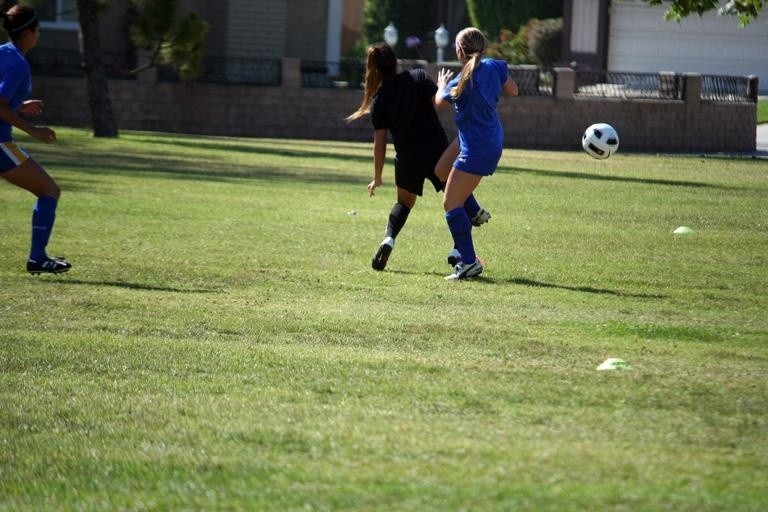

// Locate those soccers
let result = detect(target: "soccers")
[582,123,619,159]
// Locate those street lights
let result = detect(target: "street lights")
[430,21,452,63]
[383,21,402,48]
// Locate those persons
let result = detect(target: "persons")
[0,4,72,277]
[345,42,492,270]
[433,27,518,281]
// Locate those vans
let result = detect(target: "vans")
[22,18,104,76]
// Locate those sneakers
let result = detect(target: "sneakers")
[471,207,492,227]
[443,257,484,281]
[448,249,462,267]
[26,256,72,276]
[370,235,395,271]
